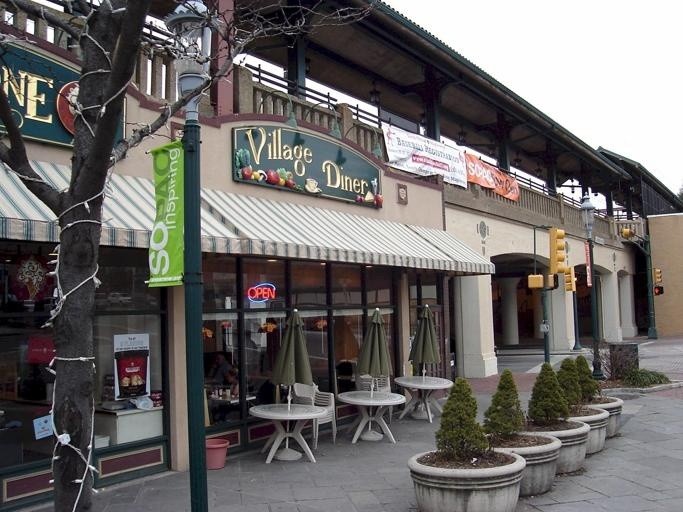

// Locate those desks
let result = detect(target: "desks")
[208,394,256,421]
[338,390,407,445]
[394,375,454,423]
[248,402,328,465]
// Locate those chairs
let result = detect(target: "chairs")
[355,367,395,425]
[293,382,337,451]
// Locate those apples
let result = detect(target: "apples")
[360,197,364,202]
[285,179,296,188]
[355,195,361,202]
[242,167,252,179]
[265,170,280,185]
[374,195,384,207]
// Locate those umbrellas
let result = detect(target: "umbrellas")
[408,304,441,411]
[356,308,394,431]
[269,309,312,447]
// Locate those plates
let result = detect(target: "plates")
[305,185,319,194]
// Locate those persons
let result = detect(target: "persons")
[208,353,231,384]
[211,368,240,422]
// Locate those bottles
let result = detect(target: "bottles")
[215,387,230,399]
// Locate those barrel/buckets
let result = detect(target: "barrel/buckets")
[206,438,231,469]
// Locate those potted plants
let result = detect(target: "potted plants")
[406,358,624,512]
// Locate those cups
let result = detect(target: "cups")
[306,178,318,191]
